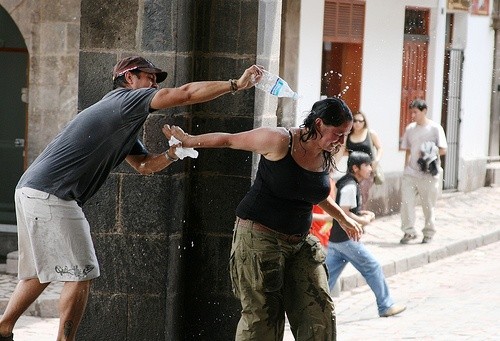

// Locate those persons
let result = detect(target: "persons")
[309,112,408,318]
[0,56,265,341]
[399,100,447,243]
[161,98,363,341]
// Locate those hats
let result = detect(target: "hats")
[112,56,168,84]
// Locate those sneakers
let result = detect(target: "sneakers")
[422,236,431,242]
[400,232,416,243]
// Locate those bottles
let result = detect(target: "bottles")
[248,68,298,99]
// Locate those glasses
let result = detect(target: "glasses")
[355,119,363,123]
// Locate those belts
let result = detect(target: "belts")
[238,218,310,245]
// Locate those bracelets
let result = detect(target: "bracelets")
[229,78,238,95]
[164,150,178,162]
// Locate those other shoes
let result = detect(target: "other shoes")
[380,304,406,317]
